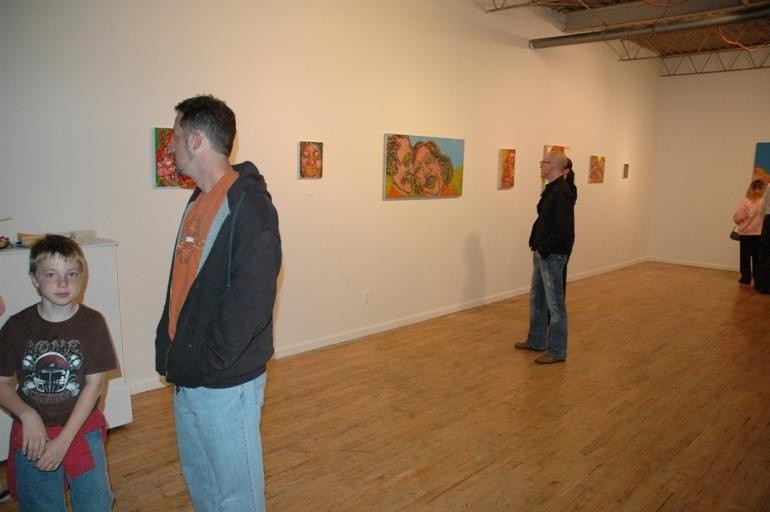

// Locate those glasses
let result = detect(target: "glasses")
[541,160,551,164]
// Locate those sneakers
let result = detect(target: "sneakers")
[515,342,546,351]
[534,355,565,363]
[739,279,751,284]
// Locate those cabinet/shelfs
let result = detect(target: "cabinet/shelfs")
[0,239,133,463]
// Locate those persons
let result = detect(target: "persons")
[501,150,515,187]
[1,234,117,510]
[733,179,770,296]
[514,150,577,365]
[154,95,281,512]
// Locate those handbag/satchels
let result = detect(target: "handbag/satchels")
[730,231,740,240]
